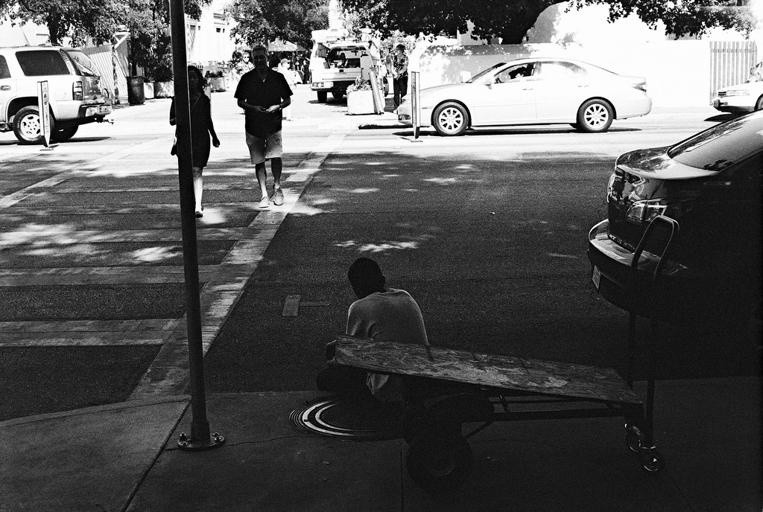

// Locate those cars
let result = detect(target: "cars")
[0,46,114,142]
[396,56,653,137]
[712,74,763,114]
[587,109,763,322]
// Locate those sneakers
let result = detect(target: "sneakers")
[258,184,284,208]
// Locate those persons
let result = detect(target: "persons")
[234,51,255,75]
[514,70,524,78]
[393,43,408,111]
[234,44,293,208]
[295,56,309,83]
[315,257,432,419]
[276,57,293,121]
[169,66,221,219]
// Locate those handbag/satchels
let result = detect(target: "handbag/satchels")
[171,142,177,155]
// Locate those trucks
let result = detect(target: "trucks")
[312,39,389,101]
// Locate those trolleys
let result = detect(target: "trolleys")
[332,215,678,473]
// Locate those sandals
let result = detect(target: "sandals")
[194,205,203,218]
